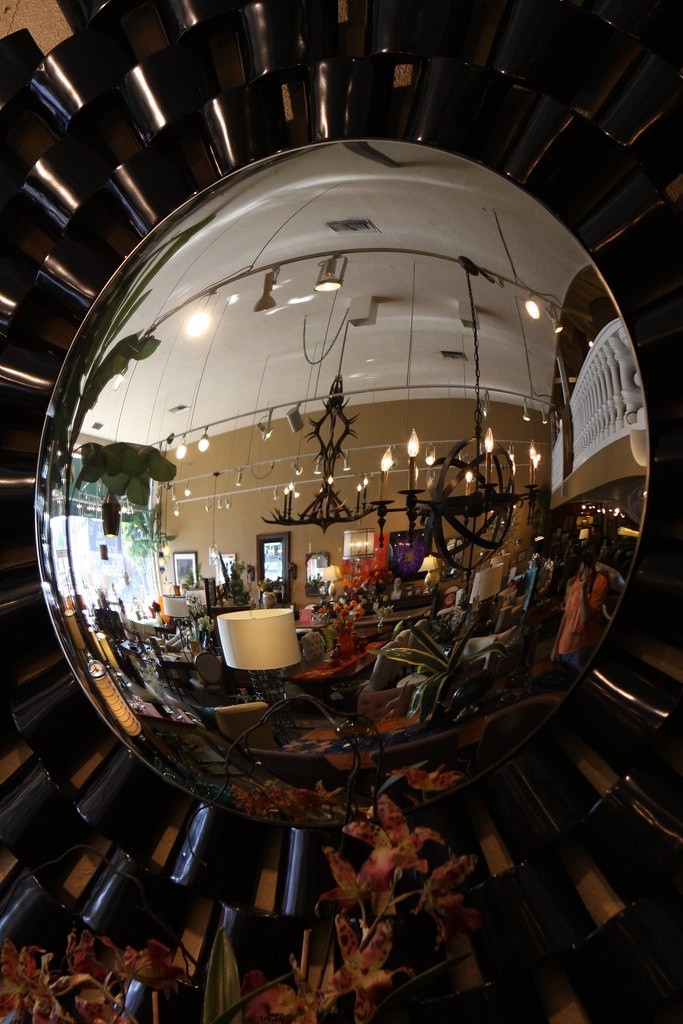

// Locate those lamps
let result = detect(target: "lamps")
[88,660,159,758]
[96,632,132,694]
[469,563,502,603]
[218,609,302,748]
[491,553,509,577]
[261,309,378,534]
[88,628,125,691]
[209,473,221,566]
[418,554,439,593]
[369,272,541,570]
[323,565,342,601]
[343,528,375,574]
[64,610,89,663]
[163,594,190,631]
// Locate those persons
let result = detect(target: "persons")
[548,540,606,671]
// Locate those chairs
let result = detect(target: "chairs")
[245,748,347,792]
[371,729,460,773]
[359,619,428,694]
[301,632,325,661]
[463,626,520,674]
[190,651,228,706]
[476,695,560,772]
[358,684,415,722]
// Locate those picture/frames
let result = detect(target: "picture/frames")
[256,533,290,603]
[388,529,428,581]
[215,553,236,587]
[173,551,199,596]
[342,532,388,594]
[517,549,527,564]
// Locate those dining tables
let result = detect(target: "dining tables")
[129,617,163,637]
[294,642,387,703]
[296,606,432,633]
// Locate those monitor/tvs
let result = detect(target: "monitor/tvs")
[95,608,127,642]
